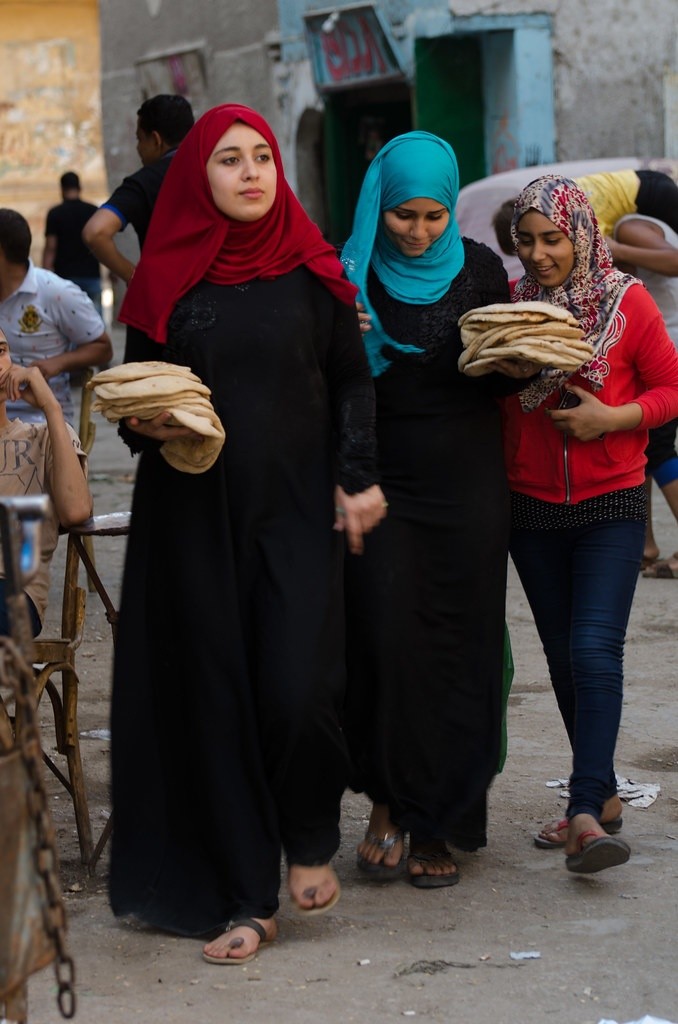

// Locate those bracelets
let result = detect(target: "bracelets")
[127,269,136,285]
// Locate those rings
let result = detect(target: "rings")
[382,502,388,508]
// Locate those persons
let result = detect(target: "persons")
[339,130,515,886]
[493,170,678,256]
[108,103,388,964]
[0,207,113,428]
[0,328,93,655]
[605,213,678,348]
[42,172,103,388]
[82,94,195,286]
[503,175,678,872]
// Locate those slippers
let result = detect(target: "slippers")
[534,814,632,873]
[201,918,279,965]
[288,861,343,918]
[357,829,462,889]
[640,550,678,579]
